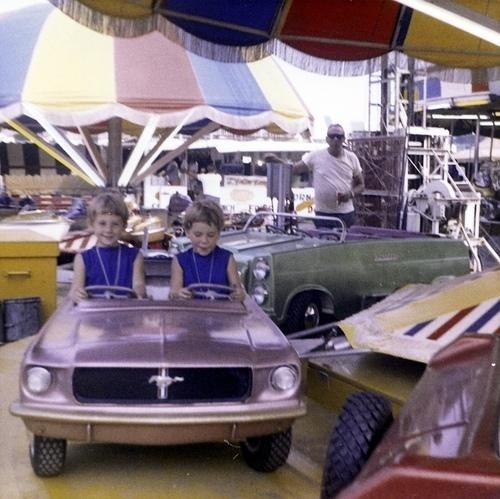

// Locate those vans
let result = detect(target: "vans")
[444,158,499,237]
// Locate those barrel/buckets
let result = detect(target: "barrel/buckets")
[3,295,39,342]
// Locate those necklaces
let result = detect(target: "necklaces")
[95,244,121,286]
[191,250,212,299]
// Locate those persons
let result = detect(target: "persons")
[262,123,365,239]
[69,191,146,304]
[167,199,246,303]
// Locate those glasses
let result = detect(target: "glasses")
[327,135,344,140]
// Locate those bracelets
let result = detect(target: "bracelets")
[350,191,354,198]
